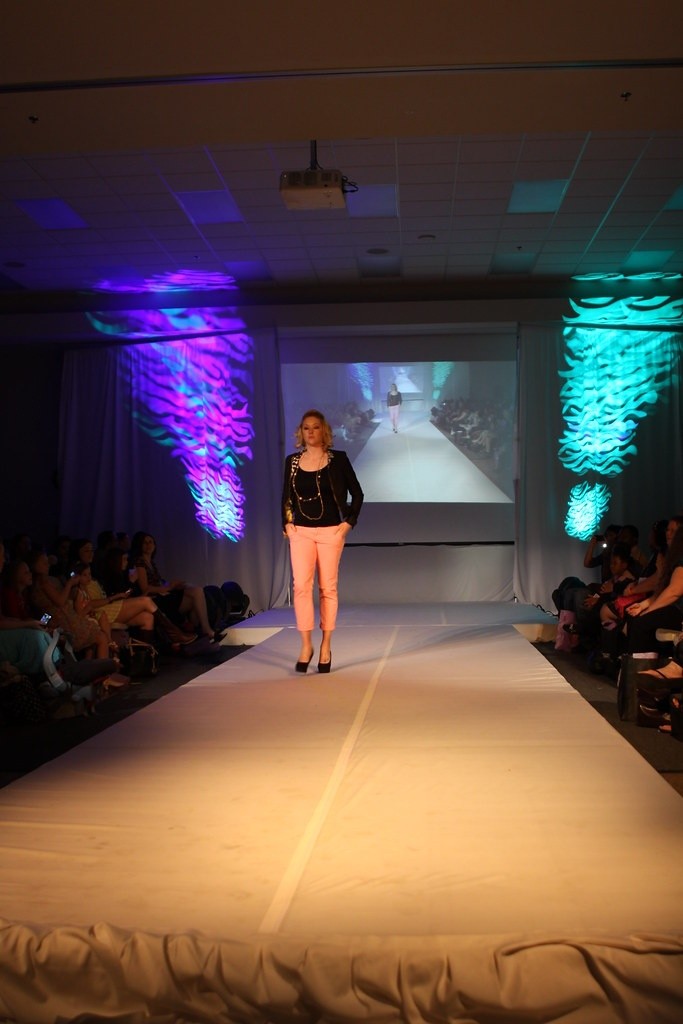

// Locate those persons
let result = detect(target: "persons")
[319,399,376,444]
[429,395,514,457]
[280,408,365,676]
[552,517,683,744]
[386,383,403,434]
[0,532,218,714]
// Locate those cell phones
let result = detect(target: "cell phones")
[594,534,605,541]
[39,612,53,628]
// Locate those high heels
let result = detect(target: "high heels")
[295,649,314,672]
[317,651,331,672]
[210,632,227,646]
[392,429,398,433]
[103,678,124,690]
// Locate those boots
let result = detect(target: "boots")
[130,628,152,651]
[152,608,198,651]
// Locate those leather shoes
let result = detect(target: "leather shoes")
[634,668,682,733]
[562,624,579,634]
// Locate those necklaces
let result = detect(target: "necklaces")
[292,450,328,520]
